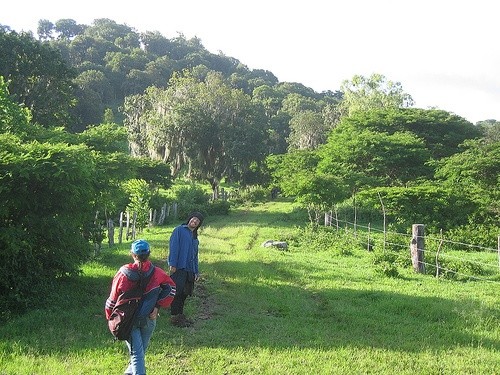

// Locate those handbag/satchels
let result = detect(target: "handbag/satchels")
[108,293,139,340]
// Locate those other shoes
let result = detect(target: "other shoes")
[170,314,193,328]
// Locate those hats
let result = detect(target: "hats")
[131,239,149,255]
[182,213,203,239]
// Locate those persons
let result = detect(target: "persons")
[104,239,175,375]
[168,212,205,328]
